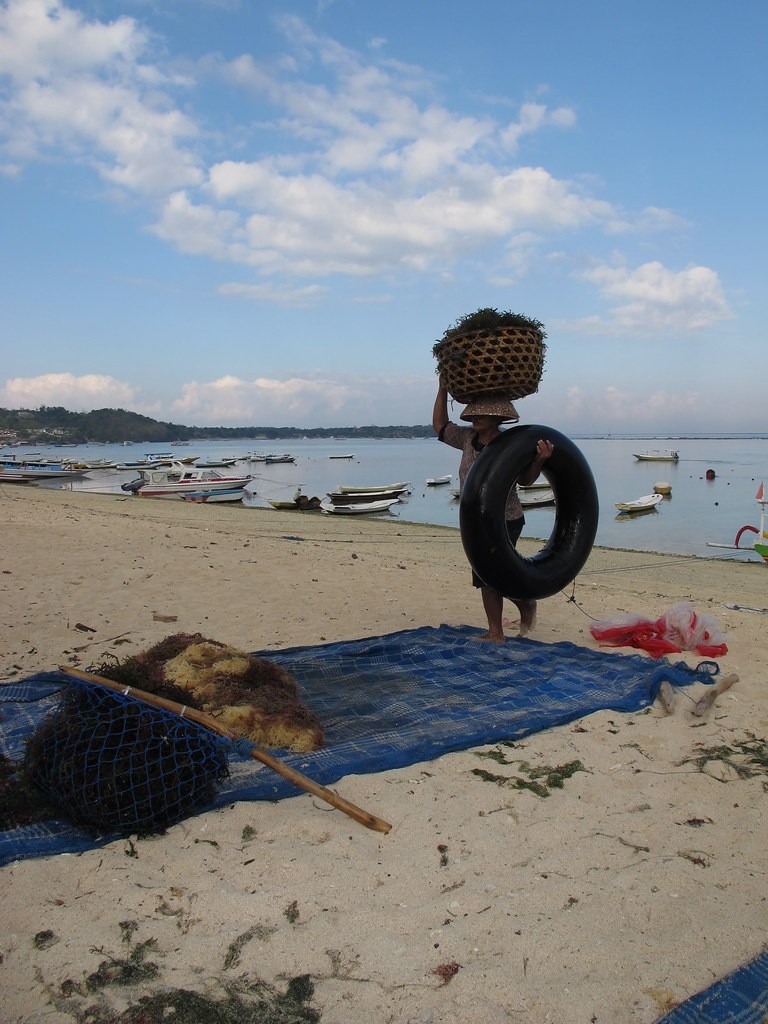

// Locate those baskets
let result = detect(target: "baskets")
[436,327,542,404]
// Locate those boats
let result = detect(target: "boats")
[269,499,322,509]
[339,482,410,493]
[518,480,550,489]
[448,489,460,496]
[326,489,408,502]
[425,474,452,485]
[0,441,295,482]
[653,480,673,494]
[632,452,679,460]
[184,489,247,503]
[614,494,663,514]
[521,497,555,505]
[121,468,255,498]
[326,499,399,514]
[329,453,355,459]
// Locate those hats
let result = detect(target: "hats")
[461,397,519,422]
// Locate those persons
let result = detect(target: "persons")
[433,381,556,644]
